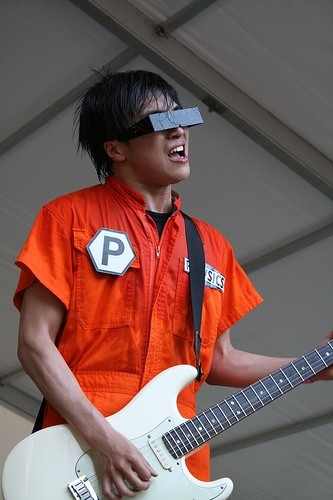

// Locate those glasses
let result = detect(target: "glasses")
[116,106,203,141]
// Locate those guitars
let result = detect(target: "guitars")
[0,339,333,500]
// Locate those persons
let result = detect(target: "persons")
[9,67,333,500]
